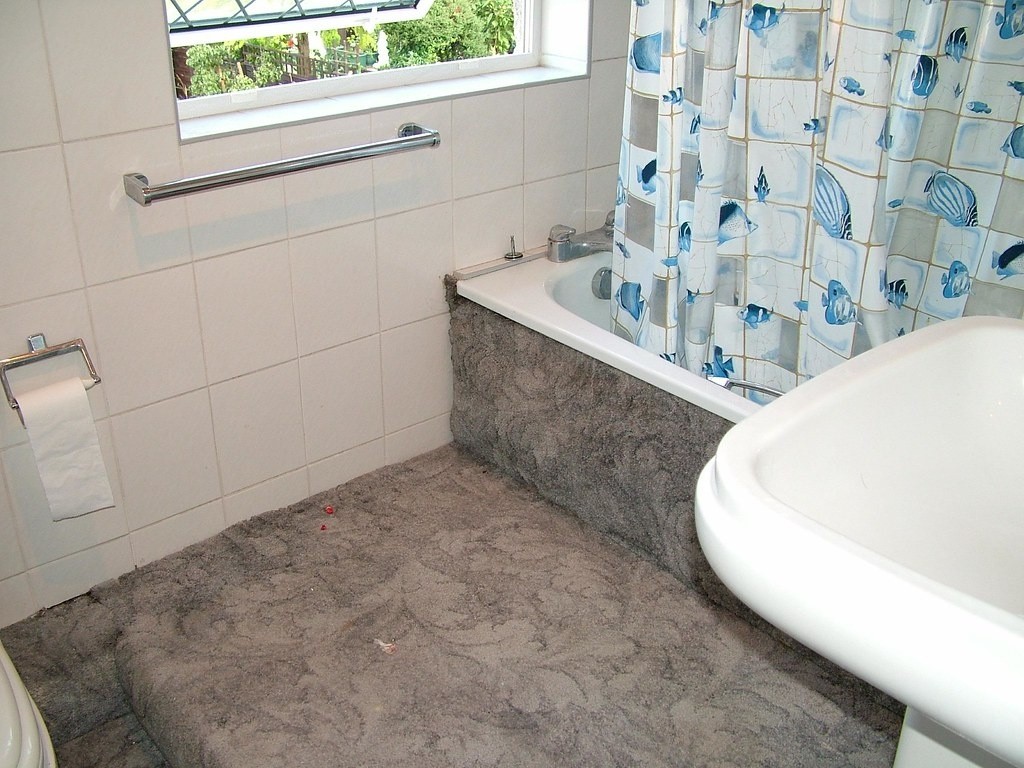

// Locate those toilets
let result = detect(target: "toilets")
[1,634,62,768]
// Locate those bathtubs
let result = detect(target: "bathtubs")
[450,243,908,719]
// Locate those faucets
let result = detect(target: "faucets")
[547,209,616,264]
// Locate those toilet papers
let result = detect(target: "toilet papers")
[15,375,118,525]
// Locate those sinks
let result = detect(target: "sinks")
[693,311,1022,768]
